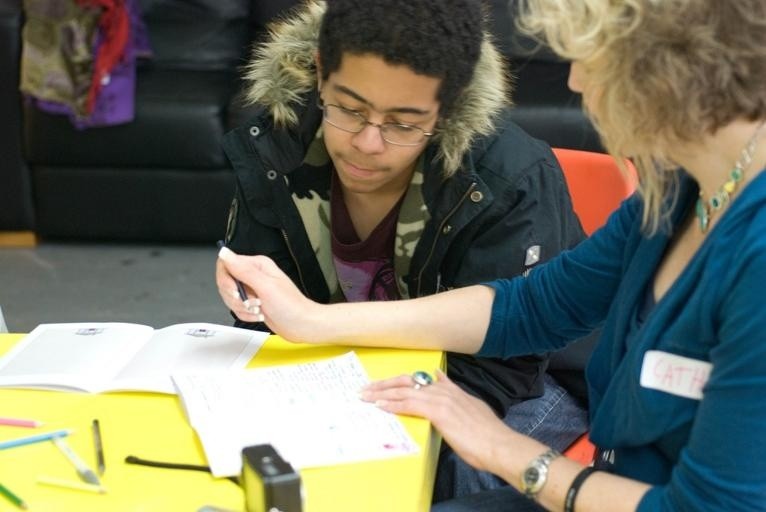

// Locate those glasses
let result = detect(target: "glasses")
[316,100,435,146]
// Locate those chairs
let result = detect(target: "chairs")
[552,145,638,465]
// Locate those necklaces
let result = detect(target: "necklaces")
[694,123,763,233]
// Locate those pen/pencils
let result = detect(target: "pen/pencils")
[0,418,38,427]
[51,436,99,485]
[0,431,68,450]
[0,484,31,511]
[38,476,107,493]
[216,240,252,315]
[93,419,106,474]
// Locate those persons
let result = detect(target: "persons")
[215,0,766,512]
[220,2,603,499]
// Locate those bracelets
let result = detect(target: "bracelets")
[564,467,602,512]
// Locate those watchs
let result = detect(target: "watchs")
[523,448,562,501]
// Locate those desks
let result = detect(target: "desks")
[0,327,449,512]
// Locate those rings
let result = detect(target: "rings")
[412,371,433,389]
